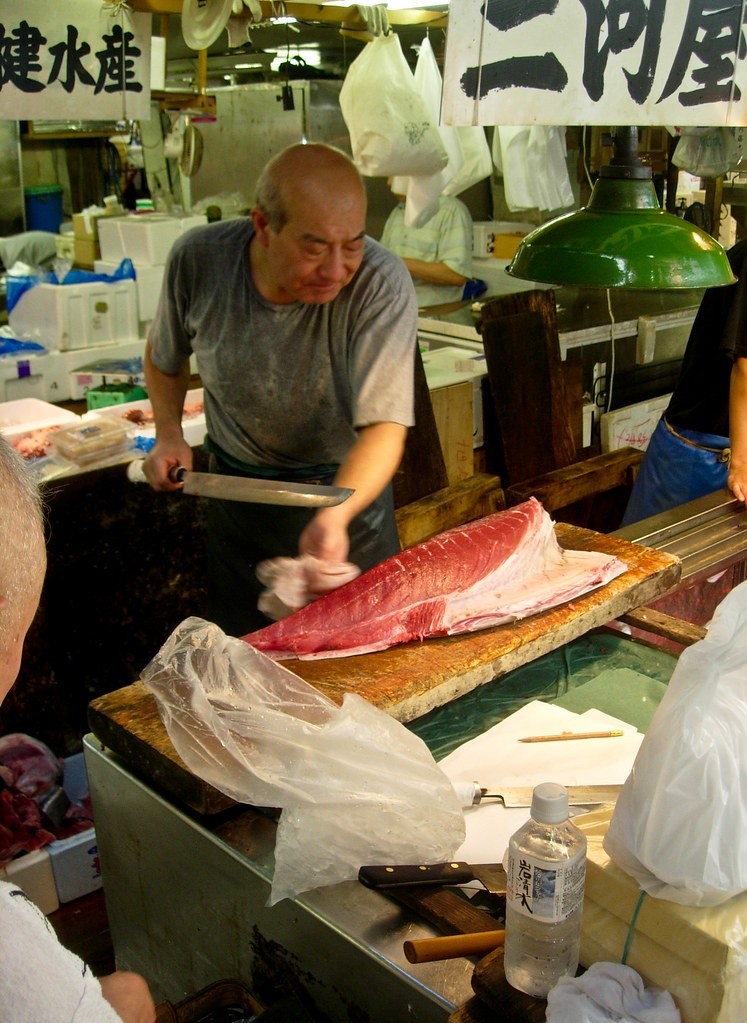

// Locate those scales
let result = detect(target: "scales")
[70,358,149,410]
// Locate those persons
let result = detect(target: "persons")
[650,134,681,214]
[378,192,468,308]
[143,142,418,636]
[0,436,157,1023]
[619,236,747,525]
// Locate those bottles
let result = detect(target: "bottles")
[503,782,587,1000]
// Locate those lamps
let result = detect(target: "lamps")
[501,124,740,290]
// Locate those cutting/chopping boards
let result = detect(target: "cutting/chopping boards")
[84,522,683,816]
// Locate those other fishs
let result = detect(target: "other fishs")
[235,495,628,662]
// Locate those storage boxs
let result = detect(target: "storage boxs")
[0,827,106,916]
[470,220,536,257]
[0,202,210,402]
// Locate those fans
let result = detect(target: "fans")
[163,123,205,176]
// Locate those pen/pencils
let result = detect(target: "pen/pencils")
[518,731,624,743]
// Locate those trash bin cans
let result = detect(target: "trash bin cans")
[23,184,64,234]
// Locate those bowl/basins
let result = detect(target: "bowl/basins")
[44,412,139,468]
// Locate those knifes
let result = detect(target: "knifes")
[128,460,356,507]
[448,782,622,811]
[358,863,508,895]
